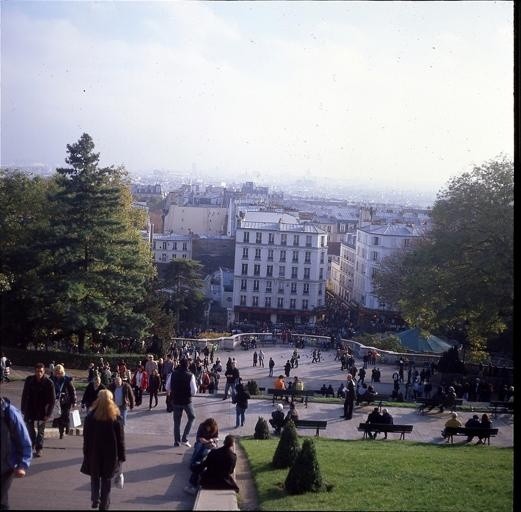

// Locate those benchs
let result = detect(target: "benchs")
[309,388,334,398]
[417,397,464,413]
[280,416,327,438]
[445,429,501,444]
[484,399,513,419]
[355,393,389,410]
[354,421,412,442]
[269,387,314,407]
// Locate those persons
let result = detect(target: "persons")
[181,326,381,410]
[441,411,462,446]
[269,402,284,434]
[381,408,394,440]
[285,401,299,427]
[481,413,494,445]
[465,414,481,443]
[48,340,224,449]
[1,353,13,383]
[367,405,382,440]
[80,388,128,511]
[253,414,271,439]
[0,395,36,510]
[20,360,58,457]
[389,352,514,410]
[184,417,220,496]
[339,373,358,421]
[194,433,241,493]
[233,385,250,428]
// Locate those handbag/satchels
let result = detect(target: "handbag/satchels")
[69,410,81,428]
[114,474,123,486]
[49,399,62,421]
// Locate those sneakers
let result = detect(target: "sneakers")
[154,404,157,407]
[36,451,43,457]
[148,407,152,410]
[65,428,69,434]
[91,501,99,508]
[174,441,182,447]
[98,504,110,510]
[60,433,63,439]
[235,424,239,428]
[180,442,191,447]
[241,424,244,426]
